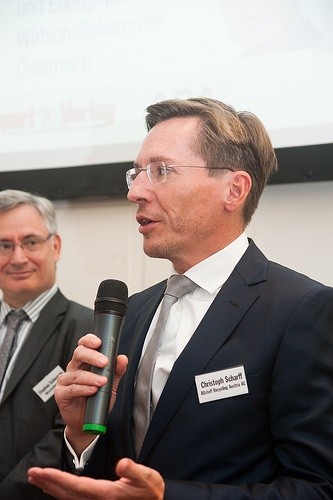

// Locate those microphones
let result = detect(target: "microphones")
[82,279,129,436]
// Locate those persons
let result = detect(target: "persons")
[0,189,95,500]
[28,97,333,499]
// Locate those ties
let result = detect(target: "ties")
[0,309,30,389]
[130,274,198,457]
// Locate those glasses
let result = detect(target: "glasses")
[0,233,53,256]
[125,160,234,189]
